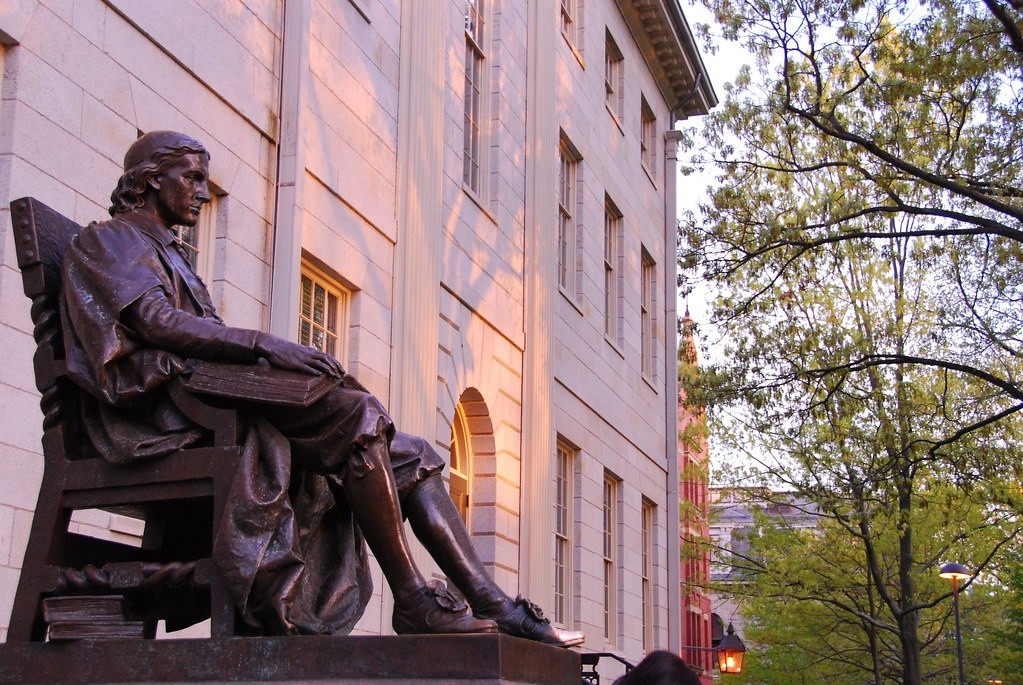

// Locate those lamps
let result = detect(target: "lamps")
[682,621,747,679]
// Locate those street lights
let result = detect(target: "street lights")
[939,563,973,684]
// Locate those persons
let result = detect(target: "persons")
[55,128,588,649]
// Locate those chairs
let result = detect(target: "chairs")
[6,197,245,639]
